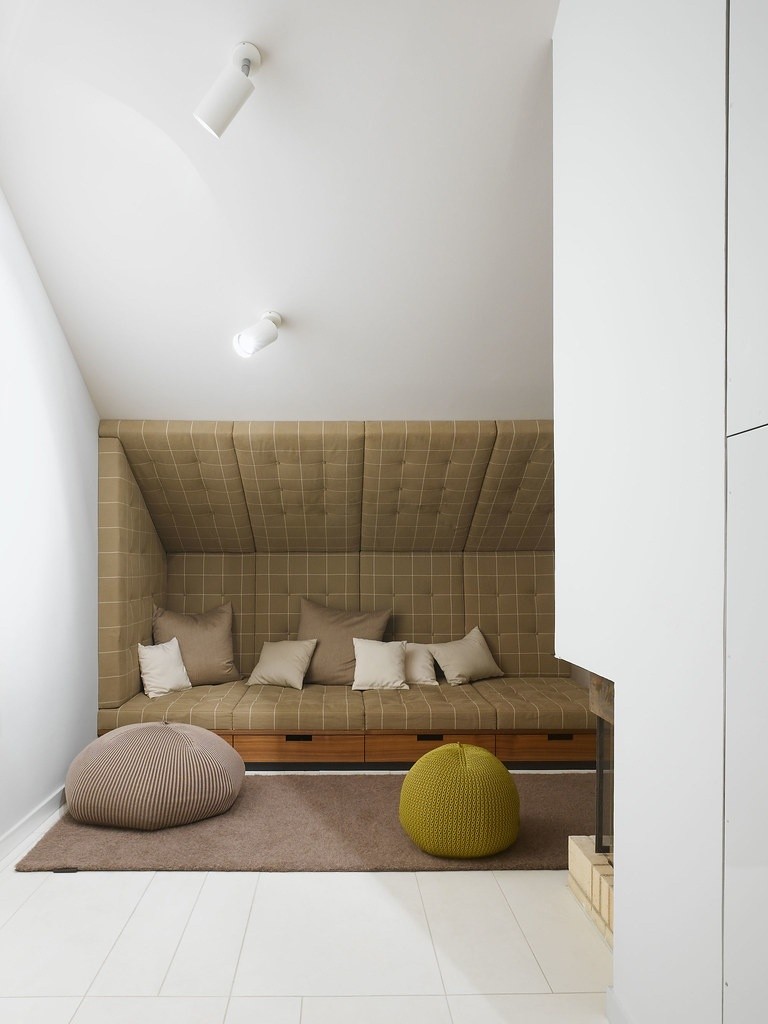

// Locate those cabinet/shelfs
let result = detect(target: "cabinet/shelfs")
[215,728,619,770]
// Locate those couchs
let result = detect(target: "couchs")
[98,418,614,731]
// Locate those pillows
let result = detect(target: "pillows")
[429,625,507,686]
[136,637,192,700]
[405,642,440,686]
[245,638,318,690]
[297,598,393,685]
[351,636,410,690]
[151,600,245,685]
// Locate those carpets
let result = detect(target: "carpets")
[13,768,614,873]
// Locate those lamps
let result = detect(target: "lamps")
[194,40,263,140]
[232,312,284,358]
